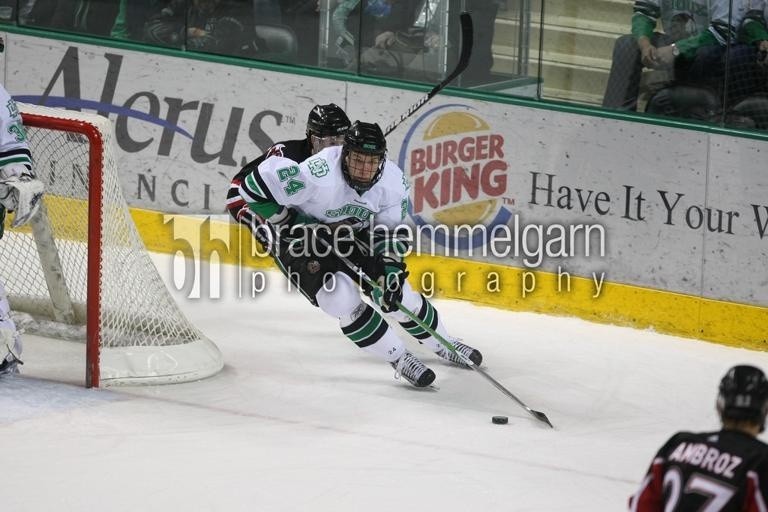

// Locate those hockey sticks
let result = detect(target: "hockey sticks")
[319,238,553,429]
[383,11,473,137]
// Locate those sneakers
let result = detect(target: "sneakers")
[434,327,482,368]
[390,349,435,388]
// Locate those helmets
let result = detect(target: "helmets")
[305,102,386,197]
[716,366,767,433]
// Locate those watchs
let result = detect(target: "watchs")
[671,42,681,57]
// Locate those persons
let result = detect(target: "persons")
[226,103,355,249]
[1,1,499,84]
[631,0,751,118]
[238,121,484,387]
[628,365,768,512]
[1,39,44,377]
[725,0,768,111]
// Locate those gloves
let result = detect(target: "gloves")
[372,262,409,314]
[279,207,332,253]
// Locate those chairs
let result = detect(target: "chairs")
[253,25,297,66]
[644,82,768,132]
